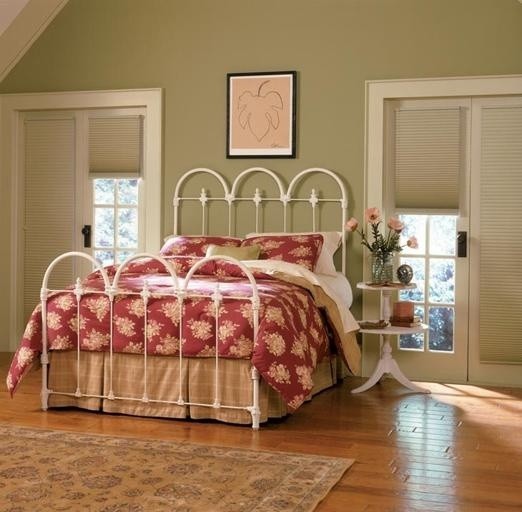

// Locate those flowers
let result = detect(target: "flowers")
[347,206,418,278]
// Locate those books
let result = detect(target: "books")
[389,315,421,328]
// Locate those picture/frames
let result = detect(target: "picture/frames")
[226,69,297,160]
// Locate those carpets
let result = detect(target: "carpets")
[1,421,361,512]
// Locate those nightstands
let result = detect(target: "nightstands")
[351,279,431,394]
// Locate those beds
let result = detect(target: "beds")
[31,166,349,429]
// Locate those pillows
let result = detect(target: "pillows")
[159,231,344,281]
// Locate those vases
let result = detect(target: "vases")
[366,252,395,283]
